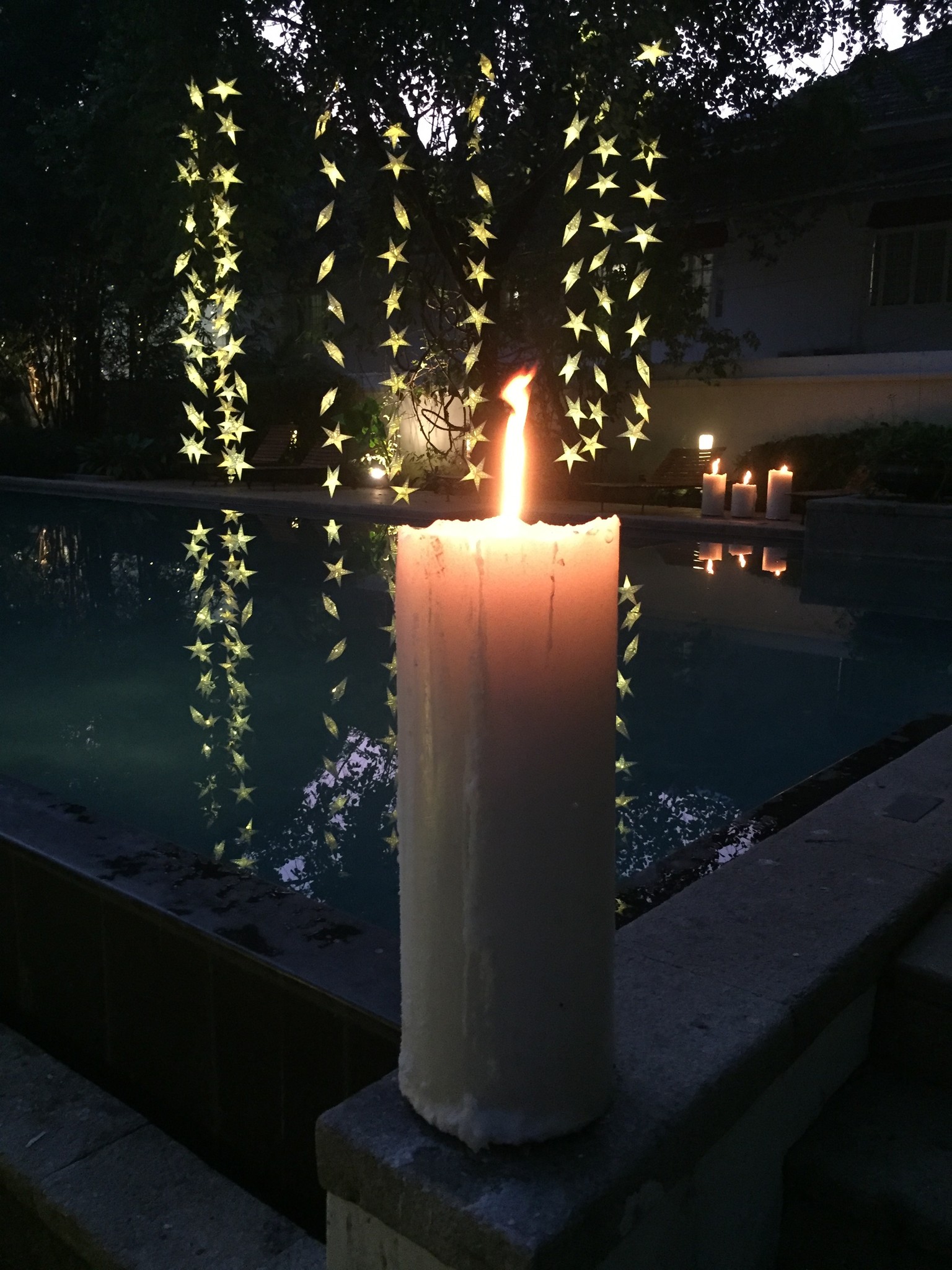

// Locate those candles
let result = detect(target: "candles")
[729,544,753,568]
[766,464,793,520]
[762,546,787,576]
[702,457,727,516]
[699,543,722,574]
[395,361,618,1147]
[731,470,757,517]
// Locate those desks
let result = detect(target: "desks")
[785,489,849,524]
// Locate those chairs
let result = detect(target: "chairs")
[585,445,726,508]
[181,422,352,492]
